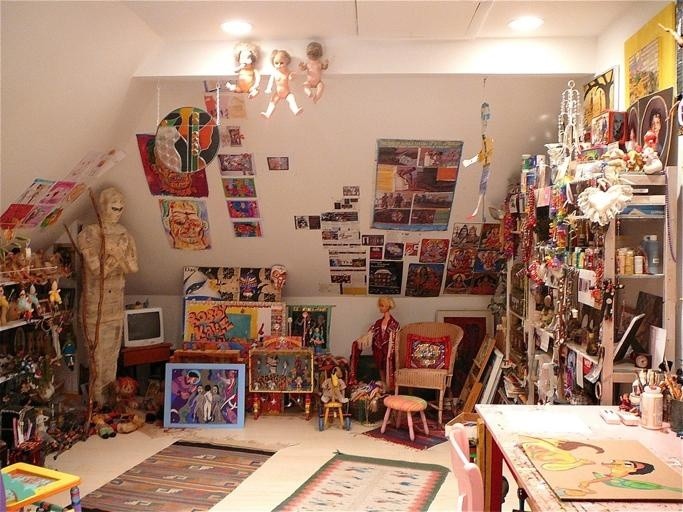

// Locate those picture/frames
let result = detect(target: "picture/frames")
[578,64,621,133]
[433,307,495,405]
[162,362,246,429]
[248,348,314,395]
[609,314,656,365]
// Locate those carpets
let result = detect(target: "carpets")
[271,451,450,512]
[60,433,297,512]
[362,422,450,452]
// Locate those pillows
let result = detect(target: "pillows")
[403,332,450,369]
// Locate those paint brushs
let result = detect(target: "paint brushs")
[632,368,683,402]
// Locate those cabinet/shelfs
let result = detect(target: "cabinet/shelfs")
[0,271,74,463]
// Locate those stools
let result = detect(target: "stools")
[382,395,431,442]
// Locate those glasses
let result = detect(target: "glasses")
[482,113,489,121]
[111,207,124,212]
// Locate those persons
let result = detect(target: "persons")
[628,120,636,143]
[146,137,197,196]
[262,49,303,119]
[369,297,401,389]
[77,188,138,423]
[169,368,240,425]
[225,42,260,99]
[299,42,329,103]
[649,107,663,157]
[160,198,208,249]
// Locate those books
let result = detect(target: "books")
[619,188,666,215]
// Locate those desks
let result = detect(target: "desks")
[473,395,683,512]
[115,343,171,391]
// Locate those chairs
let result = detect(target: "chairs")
[440,424,493,512]
[395,320,464,423]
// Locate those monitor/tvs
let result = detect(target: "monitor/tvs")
[123,307,164,347]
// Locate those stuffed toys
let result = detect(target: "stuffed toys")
[77,377,165,443]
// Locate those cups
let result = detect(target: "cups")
[670,400,683,433]
